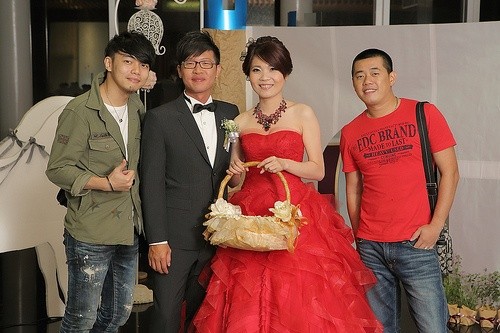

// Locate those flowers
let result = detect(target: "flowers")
[220,118,240,152]
[206,197,242,221]
[268,200,303,222]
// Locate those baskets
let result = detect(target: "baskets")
[202,161,306,252]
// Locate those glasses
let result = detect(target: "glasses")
[181,60,217,69]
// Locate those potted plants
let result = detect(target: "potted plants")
[444,254,500,327]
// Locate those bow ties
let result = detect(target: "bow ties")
[182,91,216,113]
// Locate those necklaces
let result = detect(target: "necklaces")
[394,96,399,110]
[103,83,127,122]
[252,98,287,132]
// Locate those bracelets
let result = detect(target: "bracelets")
[105,176,114,193]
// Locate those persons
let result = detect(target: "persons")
[340,49,458,333]
[0,96,102,321]
[138,30,239,333]
[46,31,145,333]
[190,36,386,333]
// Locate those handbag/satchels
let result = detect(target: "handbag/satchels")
[434,226,454,275]
